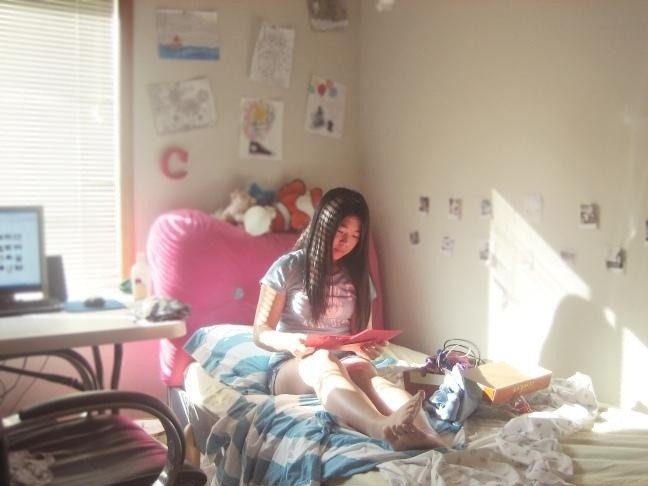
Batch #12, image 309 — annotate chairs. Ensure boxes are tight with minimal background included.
[0,350,207,486]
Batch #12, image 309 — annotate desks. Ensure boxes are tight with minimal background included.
[0,298,187,419]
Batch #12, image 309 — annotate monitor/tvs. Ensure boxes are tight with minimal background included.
[0,206,44,304]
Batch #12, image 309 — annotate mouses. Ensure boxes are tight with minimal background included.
[84,298,105,308]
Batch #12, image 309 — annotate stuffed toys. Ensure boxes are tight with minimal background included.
[216,175,323,235]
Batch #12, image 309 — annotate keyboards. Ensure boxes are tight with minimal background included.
[0,297,64,316]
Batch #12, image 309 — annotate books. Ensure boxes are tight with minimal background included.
[304,325,401,347]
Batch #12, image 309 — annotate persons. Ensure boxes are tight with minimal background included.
[251,187,448,452]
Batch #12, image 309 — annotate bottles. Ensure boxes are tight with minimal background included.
[131,252,150,300]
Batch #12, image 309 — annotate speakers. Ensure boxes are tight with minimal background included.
[45,255,68,304]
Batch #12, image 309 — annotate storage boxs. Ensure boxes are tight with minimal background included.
[403,358,553,405]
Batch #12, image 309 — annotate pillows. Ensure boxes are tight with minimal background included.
[183,322,272,394]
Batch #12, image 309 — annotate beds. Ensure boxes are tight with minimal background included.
[166,341,648,486]
